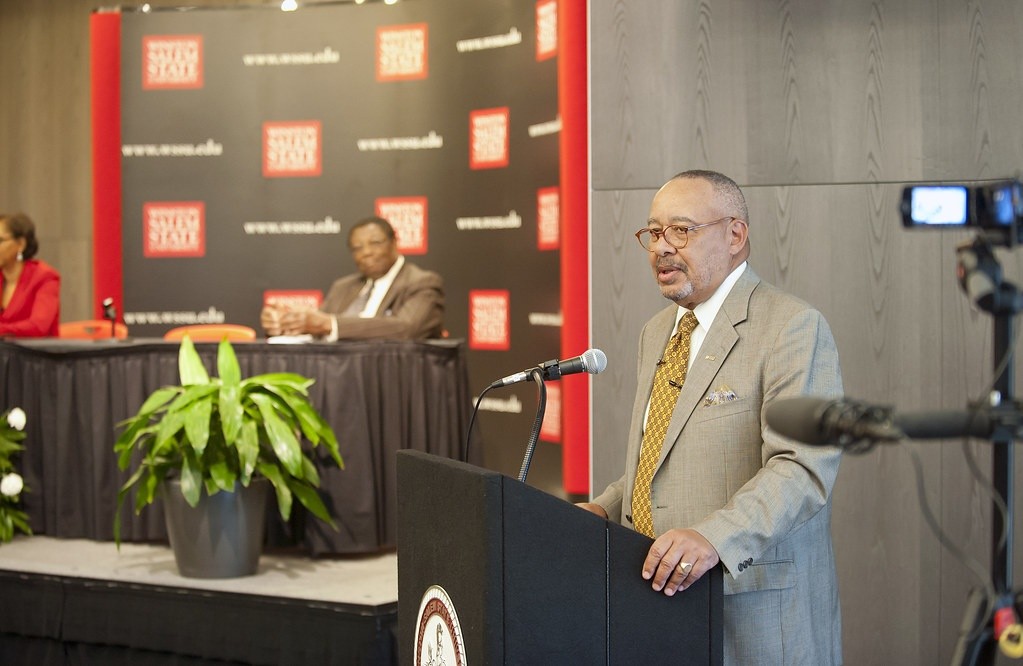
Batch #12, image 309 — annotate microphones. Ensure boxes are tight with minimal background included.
[491,349,608,389]
[766,395,902,447]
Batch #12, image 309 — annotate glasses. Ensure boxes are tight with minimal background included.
[634,216,736,252]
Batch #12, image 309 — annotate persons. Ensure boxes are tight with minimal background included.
[575,170,845,666]
[0,211,61,340]
[260,215,448,344]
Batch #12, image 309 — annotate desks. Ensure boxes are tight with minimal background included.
[1,337,479,563]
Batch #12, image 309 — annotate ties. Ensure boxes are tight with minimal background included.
[631,309,699,540]
[340,277,376,318]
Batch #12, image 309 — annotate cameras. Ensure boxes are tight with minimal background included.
[898,179,1023,230]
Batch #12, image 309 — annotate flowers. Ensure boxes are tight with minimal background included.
[0,407,33,544]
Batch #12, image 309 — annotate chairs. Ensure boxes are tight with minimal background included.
[60,320,128,339]
[166,324,256,341]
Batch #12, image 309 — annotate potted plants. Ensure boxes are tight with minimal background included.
[115,333,344,578]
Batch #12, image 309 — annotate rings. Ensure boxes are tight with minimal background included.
[678,562,692,569]
